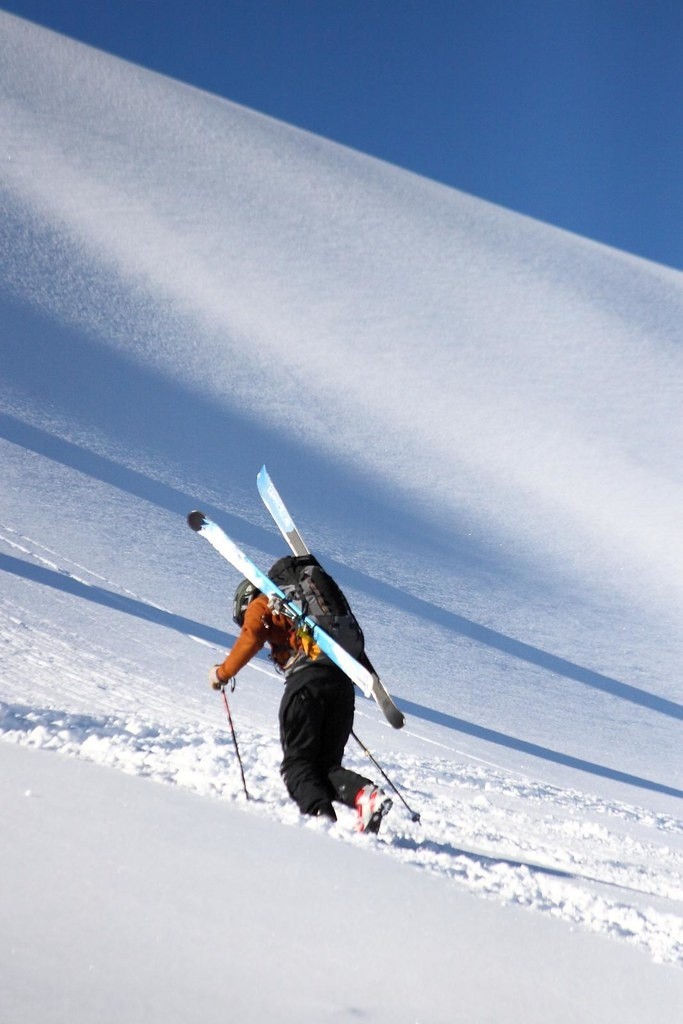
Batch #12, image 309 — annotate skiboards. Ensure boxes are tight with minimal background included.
[186,507,375,701]
[255,463,408,731]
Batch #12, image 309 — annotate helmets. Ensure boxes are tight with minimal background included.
[233,578,262,629]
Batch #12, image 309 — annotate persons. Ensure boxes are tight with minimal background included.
[209,579,393,836]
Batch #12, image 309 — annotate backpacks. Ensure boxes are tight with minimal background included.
[266,554,364,671]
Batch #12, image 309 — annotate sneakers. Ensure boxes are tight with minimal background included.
[354,785,392,835]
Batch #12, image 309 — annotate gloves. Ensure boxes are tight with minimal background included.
[209,665,228,691]
